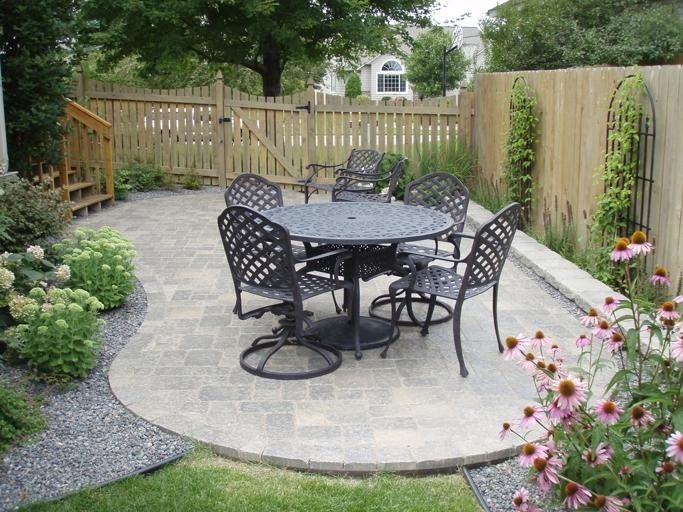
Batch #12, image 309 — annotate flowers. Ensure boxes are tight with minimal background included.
[495,229,683,511]
